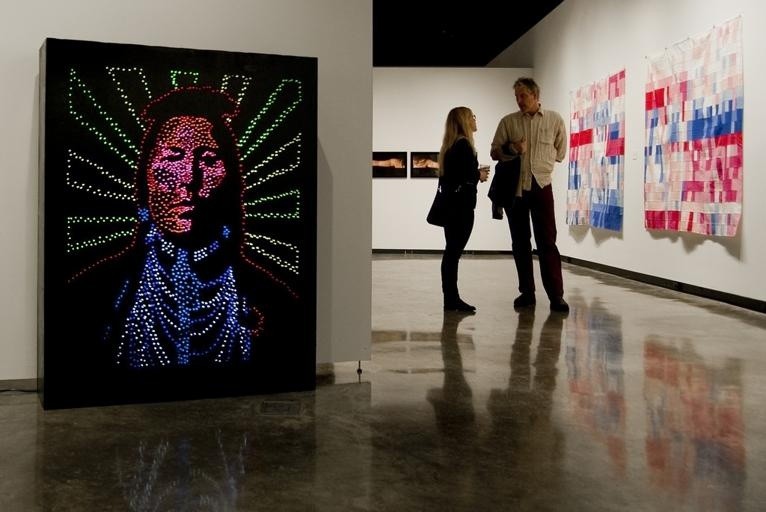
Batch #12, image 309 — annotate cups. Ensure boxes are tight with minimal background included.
[480,164,490,170]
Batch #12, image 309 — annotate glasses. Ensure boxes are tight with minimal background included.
[472,114,476,120]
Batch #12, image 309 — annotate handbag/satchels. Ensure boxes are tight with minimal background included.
[426,188,458,226]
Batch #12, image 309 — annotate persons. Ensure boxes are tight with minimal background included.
[489,78,569,313]
[427,107,490,311]
[94,87,298,403]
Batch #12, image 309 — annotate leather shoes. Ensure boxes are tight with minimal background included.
[550,297,569,313]
[444,293,476,312]
[514,293,536,306]
